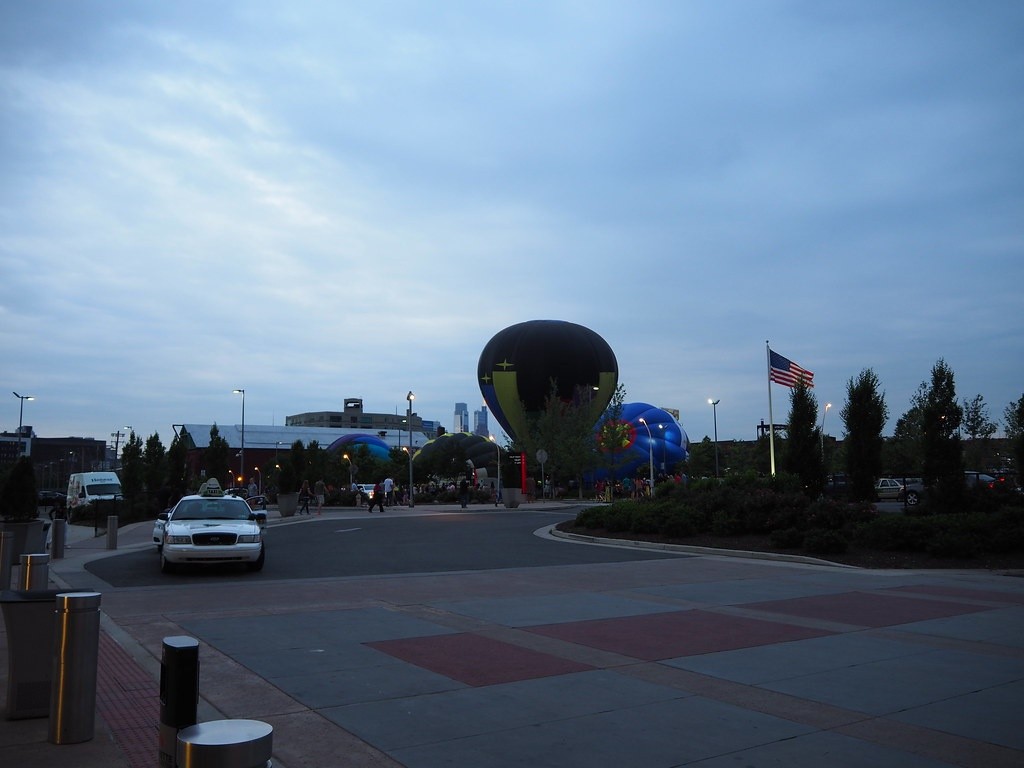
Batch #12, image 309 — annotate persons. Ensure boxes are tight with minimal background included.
[384,475,410,507]
[441,482,456,493]
[49,503,67,522]
[314,475,330,516]
[490,481,496,504]
[595,477,647,501]
[544,475,552,494]
[460,478,470,508]
[352,479,358,491]
[411,481,427,496]
[247,478,258,511]
[298,480,314,515]
[656,473,688,487]
[368,481,385,513]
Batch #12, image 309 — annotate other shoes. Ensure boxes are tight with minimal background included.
[299,512,303,515]
[307,512,311,515]
[380,510,386,512]
[367,509,373,513]
[461,506,468,509]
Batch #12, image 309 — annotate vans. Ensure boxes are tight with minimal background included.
[66,472,127,525]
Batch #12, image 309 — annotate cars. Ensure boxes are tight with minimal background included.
[38,491,68,506]
[152,503,176,550]
[875,479,907,501]
[358,484,388,499]
[965,472,1000,489]
[161,478,269,573]
[223,488,250,498]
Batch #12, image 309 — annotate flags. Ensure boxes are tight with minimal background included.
[770,349,815,390]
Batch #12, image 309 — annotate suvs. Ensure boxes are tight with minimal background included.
[898,479,933,506]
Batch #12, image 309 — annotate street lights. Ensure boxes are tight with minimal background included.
[254,467,261,496]
[228,470,235,494]
[399,419,406,451]
[489,434,501,501]
[708,398,720,478]
[343,454,353,485]
[233,389,246,488]
[658,424,669,477]
[406,391,417,508]
[640,417,654,498]
[12,391,36,462]
[275,441,282,471]
[588,386,600,488]
[821,402,833,468]
